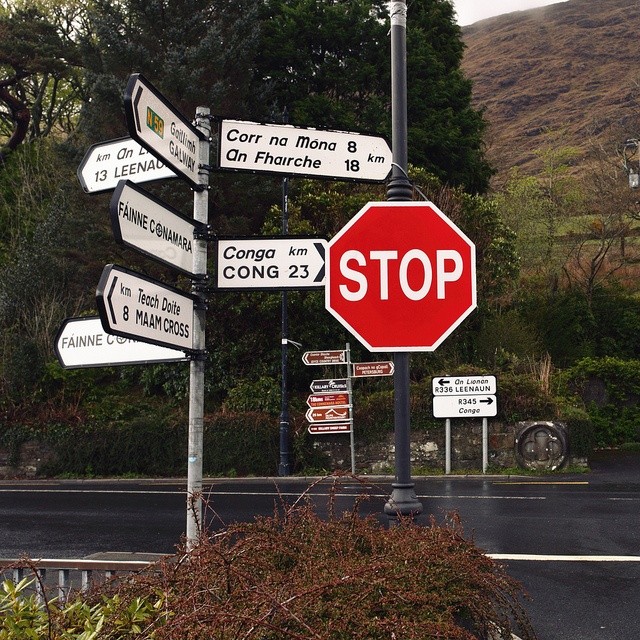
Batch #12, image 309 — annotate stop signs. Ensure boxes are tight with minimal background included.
[325,201,476,353]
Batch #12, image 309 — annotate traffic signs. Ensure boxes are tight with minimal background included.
[96,264,194,352]
[305,409,348,423]
[351,361,395,377]
[77,136,178,194]
[431,375,496,396]
[433,396,497,418]
[306,394,350,409]
[218,119,392,182]
[53,316,189,370]
[216,235,331,291]
[310,379,348,394]
[308,423,350,434]
[123,73,200,191]
[302,350,345,365]
[111,179,193,277]
